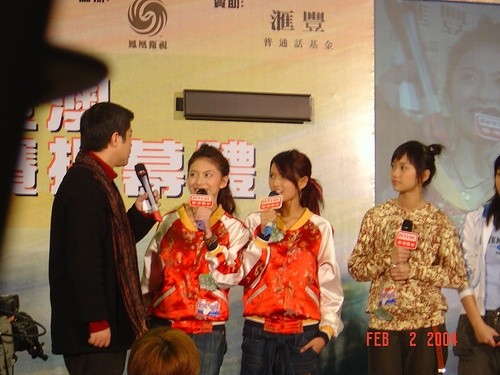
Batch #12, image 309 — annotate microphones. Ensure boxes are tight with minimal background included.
[134,163,162,222]
[189,188,213,231]
[260,191,283,240]
[395,219,418,250]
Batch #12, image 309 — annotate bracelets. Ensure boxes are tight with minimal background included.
[204,234,217,246]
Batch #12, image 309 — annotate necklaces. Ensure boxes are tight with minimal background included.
[450,152,493,201]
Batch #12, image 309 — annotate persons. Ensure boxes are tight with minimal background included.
[421,25,500,226]
[48,101,162,375]
[126,326,200,375]
[237,150,344,375]
[347,140,465,374]
[137,144,253,375]
[453,155,500,375]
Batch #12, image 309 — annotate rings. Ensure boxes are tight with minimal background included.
[394,264,396,269]
